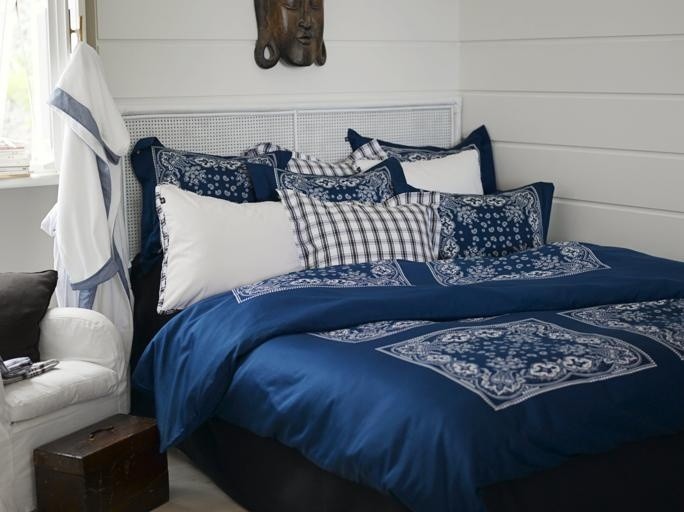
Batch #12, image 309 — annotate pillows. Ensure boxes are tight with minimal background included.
[248,138,389,179]
[0,271,57,363]
[423,181,555,257]
[241,155,410,206]
[367,143,486,196]
[340,122,498,196]
[270,184,445,270]
[131,135,293,269]
[150,181,303,316]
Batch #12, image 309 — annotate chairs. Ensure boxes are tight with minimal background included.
[0,306,125,512]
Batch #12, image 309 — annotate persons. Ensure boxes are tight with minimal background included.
[252,0,328,69]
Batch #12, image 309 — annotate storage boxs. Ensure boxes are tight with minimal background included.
[33,414,169,512]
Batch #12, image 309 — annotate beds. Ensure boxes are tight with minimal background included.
[120,96,684,512]
